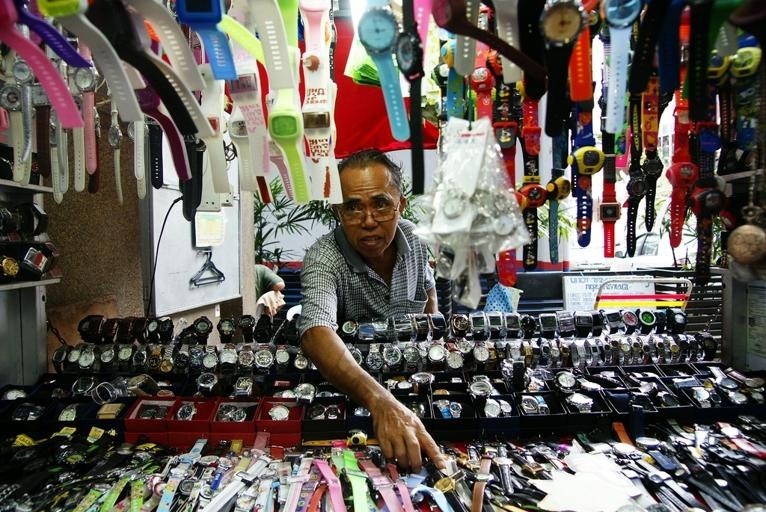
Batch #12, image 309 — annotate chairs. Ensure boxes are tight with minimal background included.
[594,278,693,314]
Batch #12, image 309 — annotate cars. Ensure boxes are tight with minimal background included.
[610,233,658,272]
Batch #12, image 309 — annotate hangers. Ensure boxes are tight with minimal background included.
[191,250,226,289]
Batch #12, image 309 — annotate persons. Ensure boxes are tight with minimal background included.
[298,150,443,474]
[254,263,285,302]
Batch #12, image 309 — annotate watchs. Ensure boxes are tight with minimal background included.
[1,1,766,285]
[1,310,766,512]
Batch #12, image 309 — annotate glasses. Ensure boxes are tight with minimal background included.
[339,201,400,226]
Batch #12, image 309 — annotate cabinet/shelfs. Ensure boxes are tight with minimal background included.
[1,70,62,292]
